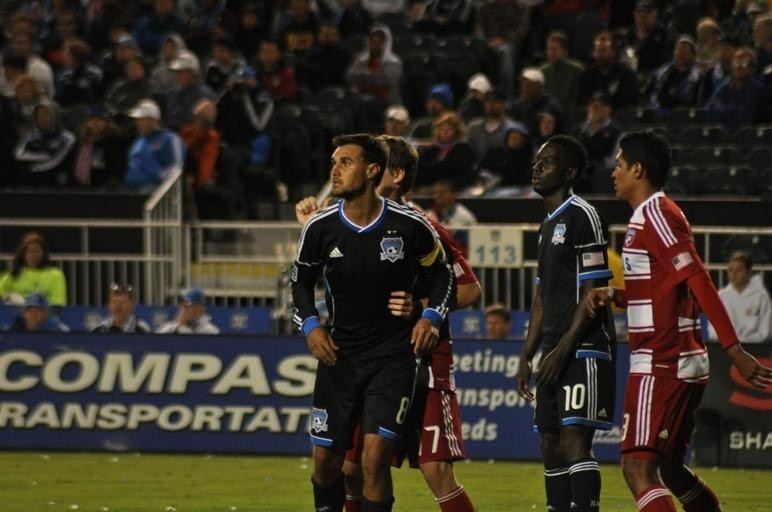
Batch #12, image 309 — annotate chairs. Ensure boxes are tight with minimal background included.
[0,0,770,209]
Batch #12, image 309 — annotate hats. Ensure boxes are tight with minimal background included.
[128,98,160,120]
[169,53,199,72]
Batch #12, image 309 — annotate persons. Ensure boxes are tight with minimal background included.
[2,0,772,228]
[8,293,69,331]
[515,136,616,512]
[486,303,510,338]
[295,135,482,512]
[155,287,220,335]
[88,282,151,334]
[291,133,455,512]
[584,132,771,512]
[2,232,66,305]
[707,251,771,342]
[604,237,625,315]
